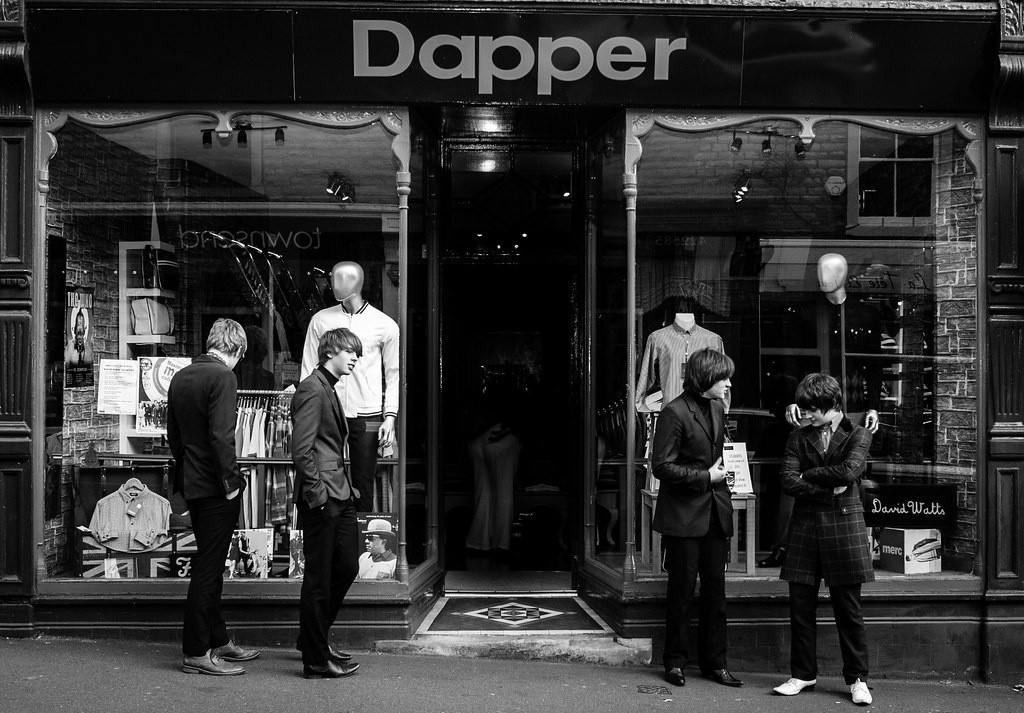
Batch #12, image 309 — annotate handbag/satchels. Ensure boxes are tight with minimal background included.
[142,244,183,291]
[130,297,174,335]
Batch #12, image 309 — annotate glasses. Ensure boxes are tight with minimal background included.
[364,535,378,541]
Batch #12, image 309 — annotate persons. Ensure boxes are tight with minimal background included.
[239,531,255,577]
[636,313,732,415]
[290,533,299,575]
[466,423,523,561]
[772,373,872,704]
[355,519,397,580]
[290,327,359,680]
[299,262,399,511]
[228,531,241,578]
[650,350,742,687]
[167,318,261,674]
[758,332,878,565]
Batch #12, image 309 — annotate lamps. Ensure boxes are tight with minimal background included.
[201,122,288,149]
[730,171,755,203]
[725,126,806,158]
[324,174,356,203]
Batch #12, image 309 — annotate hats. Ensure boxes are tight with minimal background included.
[361,519,395,537]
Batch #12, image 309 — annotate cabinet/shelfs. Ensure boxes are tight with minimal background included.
[117,240,178,453]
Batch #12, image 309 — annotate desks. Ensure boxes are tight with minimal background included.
[640,489,757,576]
[405,486,568,570]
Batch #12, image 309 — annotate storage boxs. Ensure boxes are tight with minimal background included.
[879,527,943,573]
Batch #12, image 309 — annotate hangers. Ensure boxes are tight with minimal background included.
[123,465,144,492]
[237,389,294,411]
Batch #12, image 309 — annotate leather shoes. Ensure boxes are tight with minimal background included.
[774,678,817,696]
[302,656,360,679]
[701,667,744,686]
[849,677,872,705]
[296,641,353,661]
[212,638,260,661]
[182,647,245,676]
[759,549,786,567]
[664,667,686,686]
